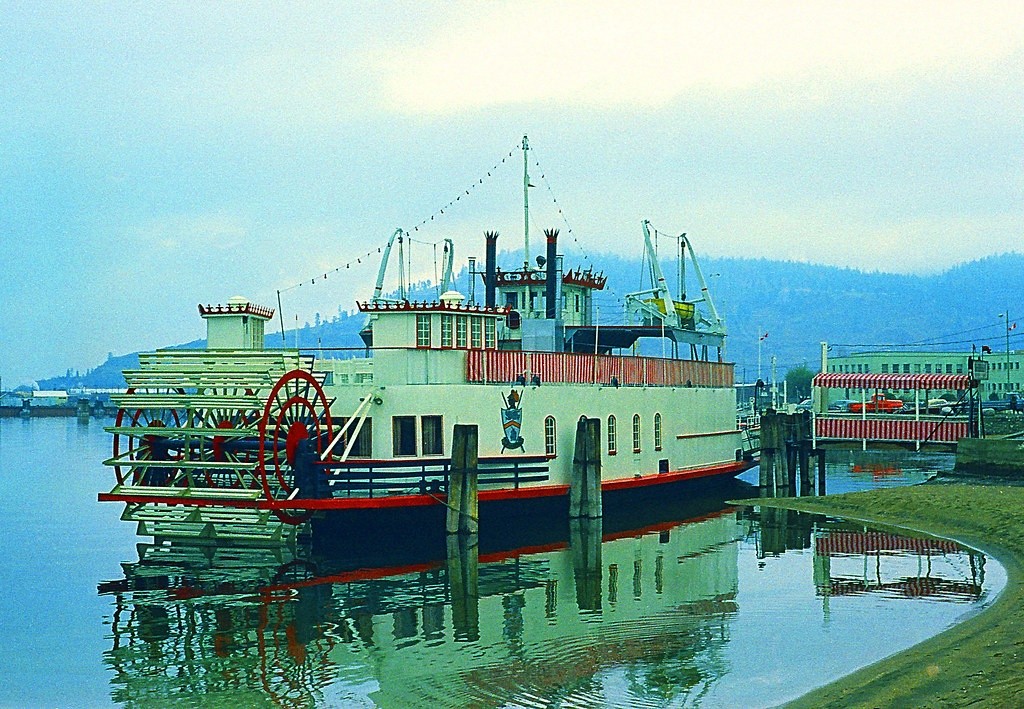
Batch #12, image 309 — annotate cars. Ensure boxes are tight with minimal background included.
[796,399,812,412]
[829,400,856,410]
[847,393,904,413]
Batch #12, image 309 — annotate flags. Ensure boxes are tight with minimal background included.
[982,346,991,354]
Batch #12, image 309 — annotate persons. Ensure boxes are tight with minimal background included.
[1010,396,1019,414]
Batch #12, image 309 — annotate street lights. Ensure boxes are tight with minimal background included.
[998,311,1009,393]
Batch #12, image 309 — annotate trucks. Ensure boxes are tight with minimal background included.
[973,392,1024,414]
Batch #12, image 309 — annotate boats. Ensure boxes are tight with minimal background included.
[96,478,762,709]
[98,136,760,542]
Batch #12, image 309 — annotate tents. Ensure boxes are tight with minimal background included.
[813,522,982,594]
[813,373,970,452]
